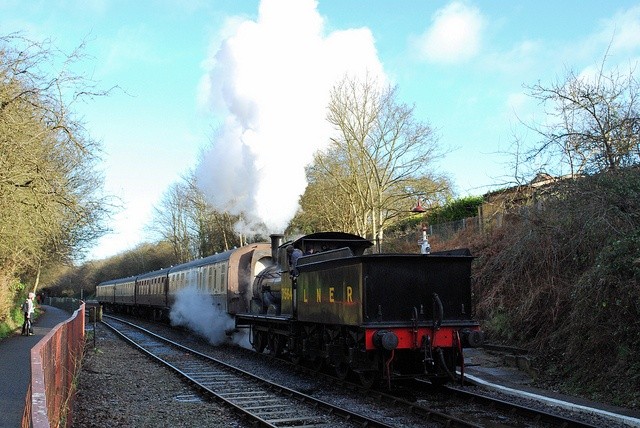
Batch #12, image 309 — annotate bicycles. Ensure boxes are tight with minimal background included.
[20,303,36,336]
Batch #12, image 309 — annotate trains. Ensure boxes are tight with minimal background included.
[95,232,483,394]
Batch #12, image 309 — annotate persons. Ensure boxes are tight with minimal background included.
[41,293,45,305]
[21,293,35,336]
[37,294,40,304]
[286,245,303,280]
[304,245,314,256]
